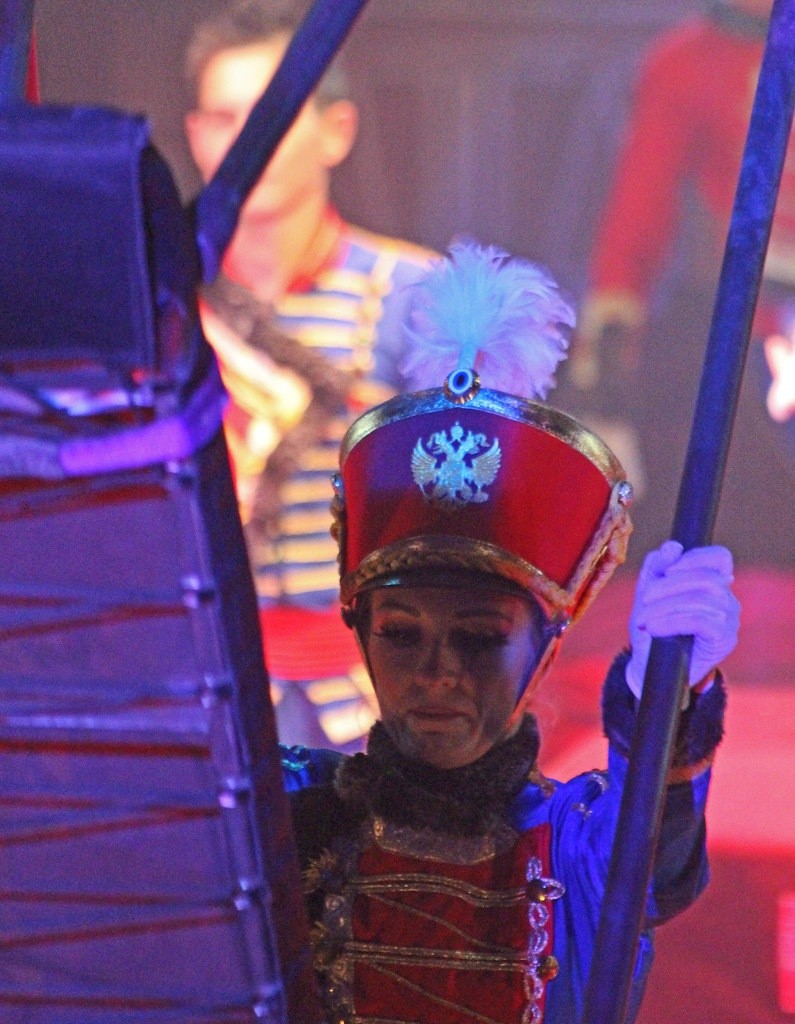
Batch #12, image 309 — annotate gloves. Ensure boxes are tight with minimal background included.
[625,541,742,713]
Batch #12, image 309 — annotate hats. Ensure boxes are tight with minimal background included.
[330,238,634,648]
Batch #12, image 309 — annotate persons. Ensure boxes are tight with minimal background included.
[180,1,477,764]
[280,369,745,1024]
[566,1,794,474]
